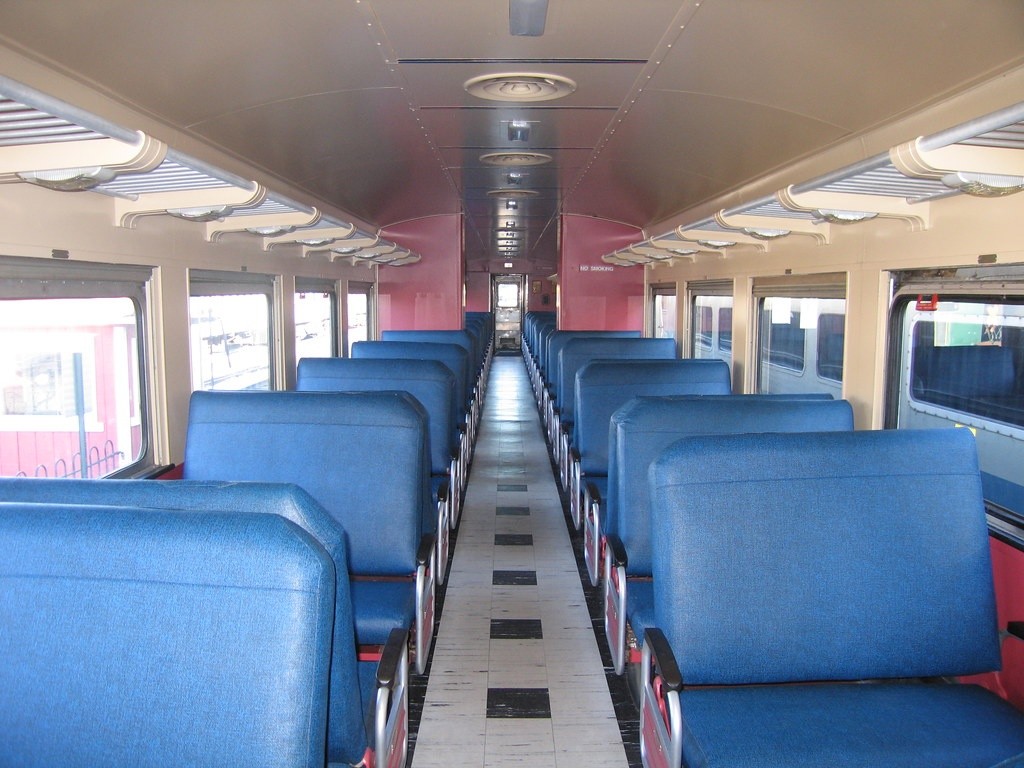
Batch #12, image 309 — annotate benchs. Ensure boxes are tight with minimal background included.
[522,311,1022,766]
[0,312,494,766]
